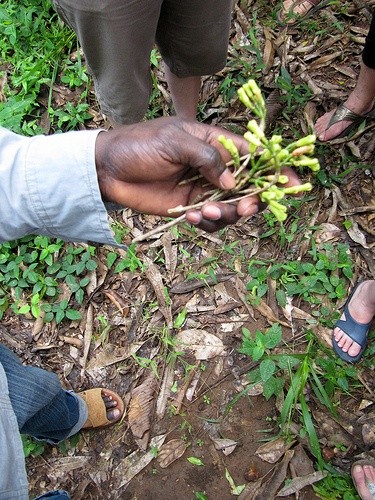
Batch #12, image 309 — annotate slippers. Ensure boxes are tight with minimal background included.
[314,102,375,143]
[332,279,375,365]
[350,459,375,500]
[74,388,125,430]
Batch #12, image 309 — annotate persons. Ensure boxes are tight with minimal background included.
[52,0,235,132]
[331,278,375,364]
[0,115,303,500]
[315,6,375,145]
[351,461,375,500]
[276,0,320,26]
